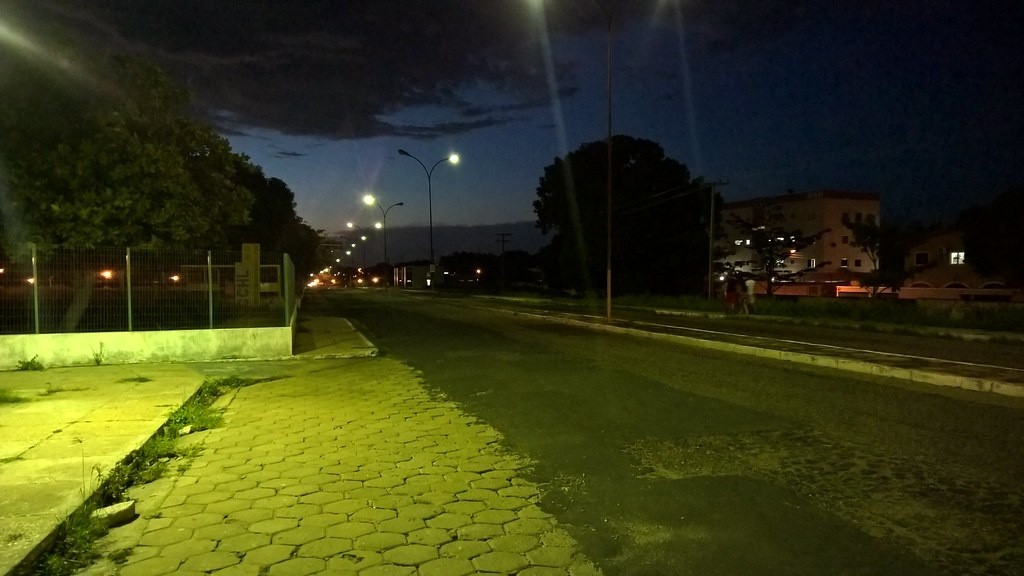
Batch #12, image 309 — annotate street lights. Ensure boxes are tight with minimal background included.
[347,222,382,272]
[364,195,404,272]
[397,149,460,290]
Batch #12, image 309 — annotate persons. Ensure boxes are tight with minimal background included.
[723,272,758,314]
